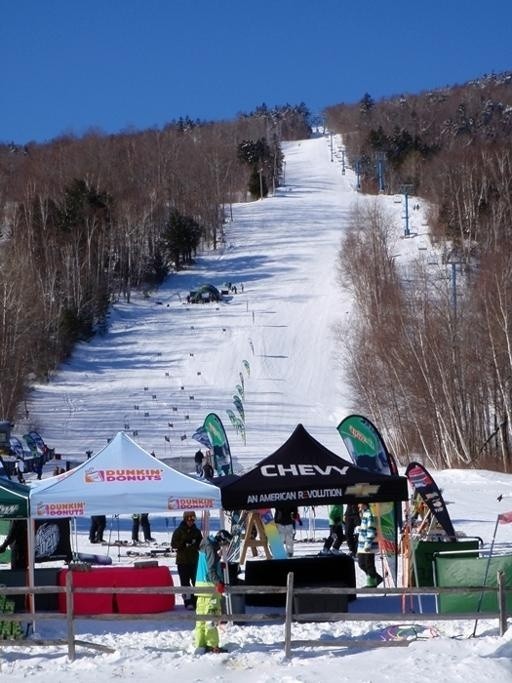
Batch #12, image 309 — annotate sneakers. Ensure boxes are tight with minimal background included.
[132,538,141,543]
[144,537,155,542]
[89,539,106,544]
[196,646,227,653]
[320,548,341,554]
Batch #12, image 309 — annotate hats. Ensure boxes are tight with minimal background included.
[184,511,196,519]
[215,529,233,543]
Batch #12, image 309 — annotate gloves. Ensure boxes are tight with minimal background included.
[215,580,226,594]
[0,544,6,554]
[354,525,361,534]
[291,512,304,525]
[364,541,372,551]
[334,517,342,525]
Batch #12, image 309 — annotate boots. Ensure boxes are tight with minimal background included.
[361,572,384,587]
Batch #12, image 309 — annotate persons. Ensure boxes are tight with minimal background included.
[35,463,43,479]
[195,449,204,473]
[170,510,206,611]
[199,458,214,480]
[1,519,29,572]
[129,512,156,542]
[224,279,245,294]
[344,502,362,557]
[191,528,234,656]
[88,515,108,544]
[292,510,303,541]
[15,456,26,482]
[411,493,430,522]
[319,504,347,554]
[273,501,298,556]
[351,503,384,587]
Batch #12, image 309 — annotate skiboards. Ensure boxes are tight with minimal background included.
[101,540,178,557]
